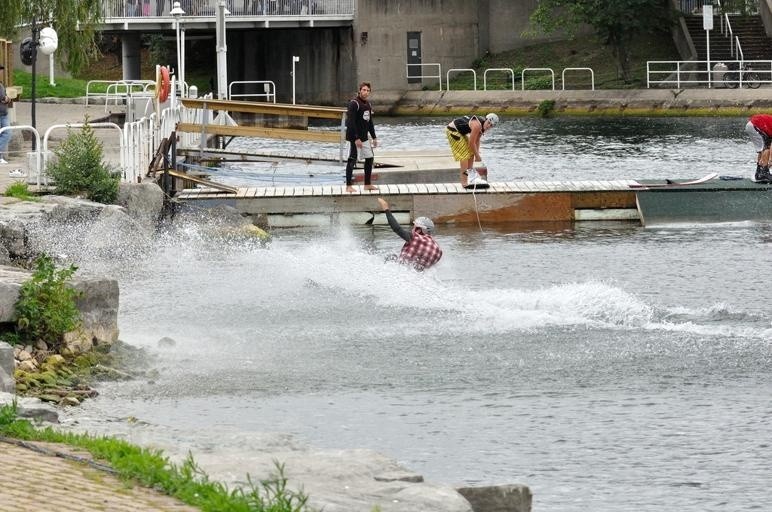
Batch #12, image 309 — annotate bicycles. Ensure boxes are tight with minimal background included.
[723,63,761,89]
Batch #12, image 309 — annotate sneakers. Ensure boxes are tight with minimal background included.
[0,158,9,164]
[7,168,29,180]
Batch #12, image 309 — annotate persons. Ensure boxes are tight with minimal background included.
[375,197,443,273]
[344,81,380,192]
[446,113,499,188]
[0,64,13,162]
[107,1,325,20]
[744,113,771,183]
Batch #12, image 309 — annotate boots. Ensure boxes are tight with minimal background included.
[755,160,771,183]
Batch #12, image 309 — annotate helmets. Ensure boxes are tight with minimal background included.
[412,215,436,237]
[485,112,499,128]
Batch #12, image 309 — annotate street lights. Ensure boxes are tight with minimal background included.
[170,1,186,98]
[20,17,58,150]
